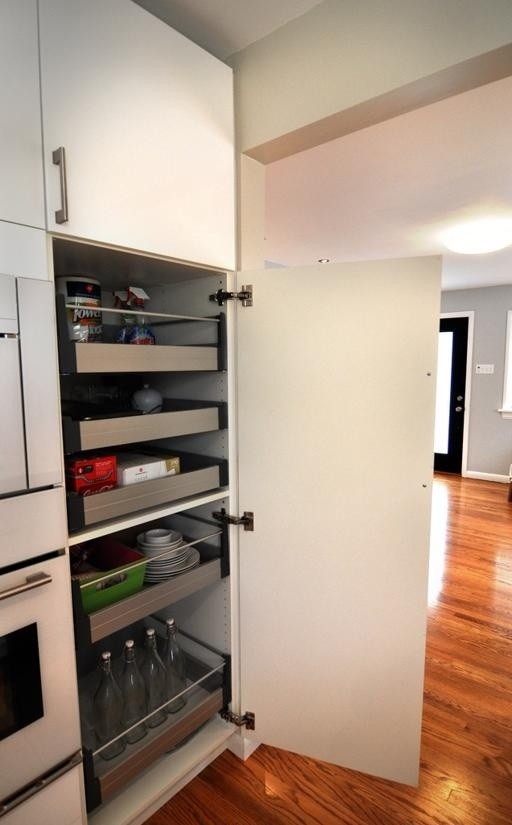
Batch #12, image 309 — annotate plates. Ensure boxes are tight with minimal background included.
[135,528,200,584]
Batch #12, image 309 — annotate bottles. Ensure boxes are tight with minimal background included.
[162,617,187,714]
[92,651,127,760]
[117,639,149,746]
[138,628,170,730]
[133,383,162,415]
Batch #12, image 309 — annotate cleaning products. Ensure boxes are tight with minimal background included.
[112,287,155,347]
